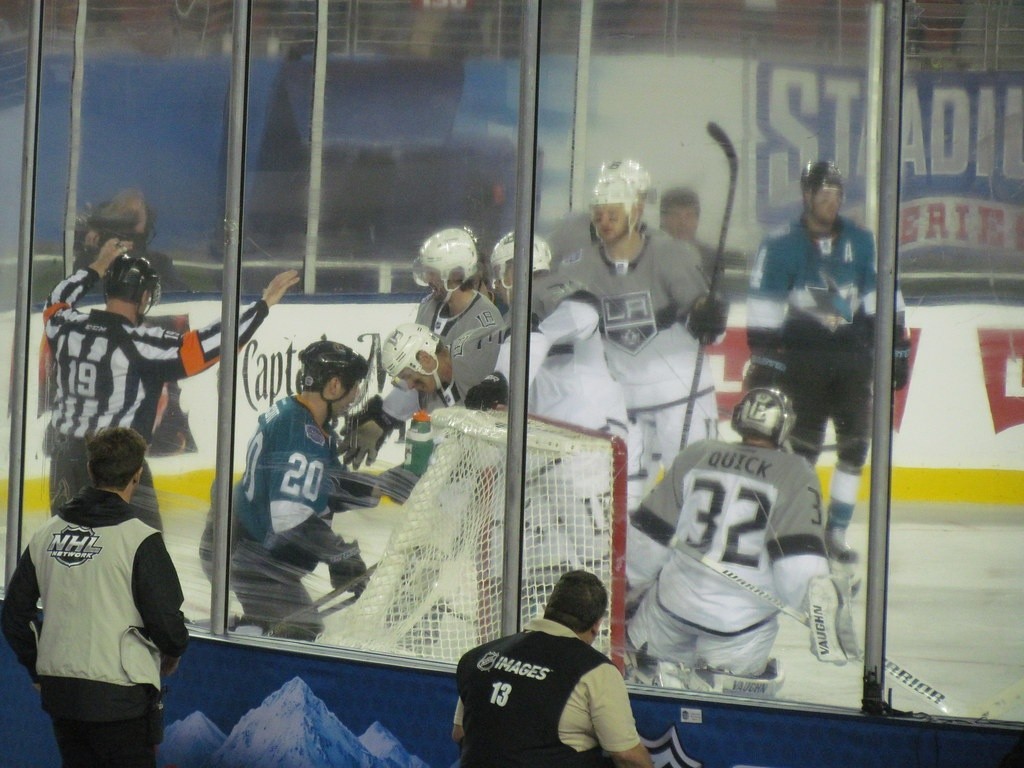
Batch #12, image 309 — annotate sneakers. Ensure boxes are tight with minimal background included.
[825,516,858,562]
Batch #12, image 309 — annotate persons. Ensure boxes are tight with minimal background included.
[555,155,729,504]
[381,228,661,687]
[453,570,653,768]
[744,159,913,576]
[0,427,189,768]
[199,339,434,643]
[42,237,301,543]
[72,187,188,302]
[634,386,860,702]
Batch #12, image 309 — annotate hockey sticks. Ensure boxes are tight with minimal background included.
[371,579,477,624]
[672,539,1023,722]
[678,120,741,450]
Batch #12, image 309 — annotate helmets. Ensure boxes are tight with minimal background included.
[420,229,478,281]
[491,231,551,274]
[802,159,843,190]
[300,341,368,383]
[381,324,441,376]
[590,175,635,213]
[102,253,159,302]
[731,387,795,446]
[601,159,649,190]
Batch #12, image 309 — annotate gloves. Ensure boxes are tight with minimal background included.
[746,326,788,391]
[889,326,912,389]
[327,541,370,598]
[338,395,405,470]
[686,289,729,345]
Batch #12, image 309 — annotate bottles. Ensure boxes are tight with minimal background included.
[403,410,434,476]
